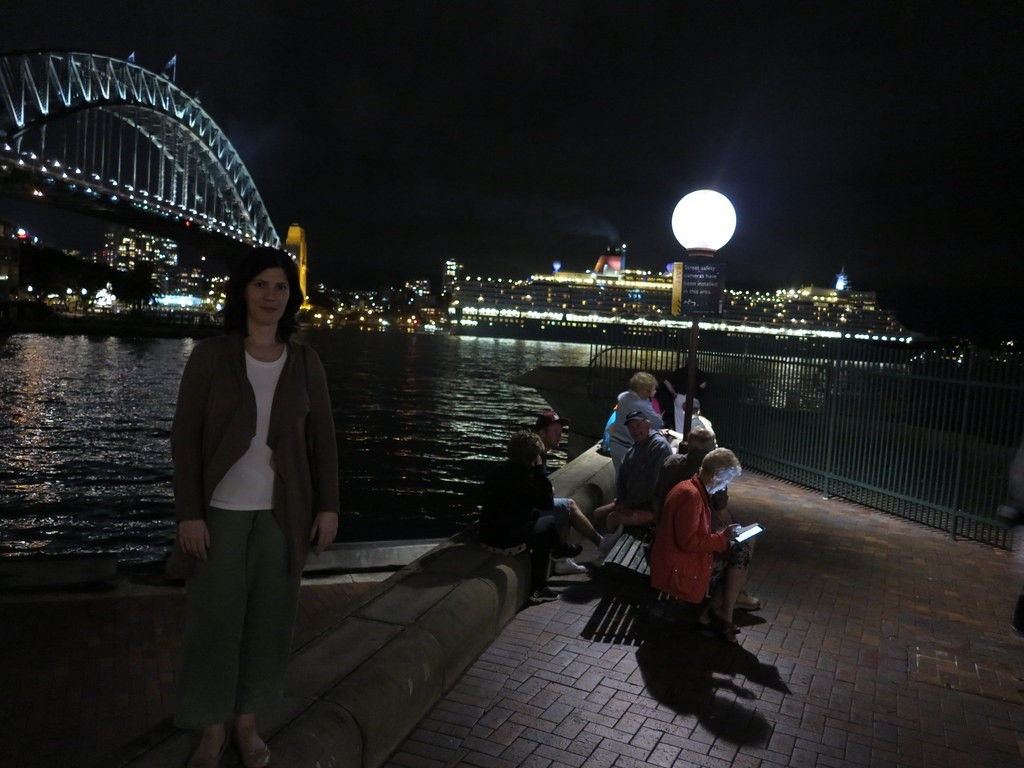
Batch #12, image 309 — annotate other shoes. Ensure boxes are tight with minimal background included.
[549,543,582,561]
[238,742,272,768]
[528,586,558,603]
[553,556,587,574]
[733,592,761,610]
[599,522,624,557]
[187,728,230,768]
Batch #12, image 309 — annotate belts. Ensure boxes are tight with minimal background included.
[676,392,685,395]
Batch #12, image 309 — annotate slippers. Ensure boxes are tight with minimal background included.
[708,605,740,644]
[695,617,717,631]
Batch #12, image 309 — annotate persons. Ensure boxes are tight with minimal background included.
[169,244,342,767]
[478,411,625,604]
[590,355,763,646]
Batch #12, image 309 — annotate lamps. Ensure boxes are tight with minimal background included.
[670,189,738,258]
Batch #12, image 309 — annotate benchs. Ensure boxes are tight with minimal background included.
[601,523,651,599]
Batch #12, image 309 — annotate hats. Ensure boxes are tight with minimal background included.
[683,400,699,410]
[623,411,647,423]
[534,412,572,434]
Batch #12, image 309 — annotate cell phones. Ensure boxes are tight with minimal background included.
[733,525,766,544]
